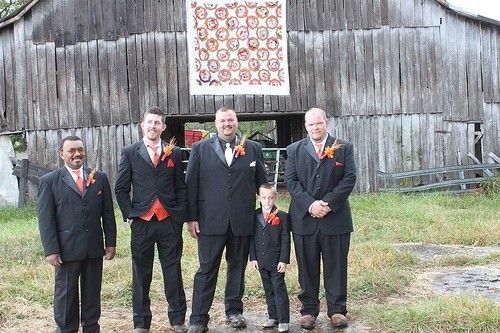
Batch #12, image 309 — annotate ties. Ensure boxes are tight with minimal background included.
[73,170,84,194]
[263,211,269,223]
[224,142,233,166]
[148,145,160,165]
[316,143,323,160]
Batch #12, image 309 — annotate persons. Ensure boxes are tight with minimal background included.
[116,107,188,333]
[248,184,291,333]
[184,107,269,333]
[37,136,117,333]
[283,108,357,330]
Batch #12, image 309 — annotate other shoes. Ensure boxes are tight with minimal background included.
[262,318,278,329]
[330,313,348,328]
[169,323,188,333]
[301,314,316,329]
[187,324,209,333]
[224,313,246,328]
[278,322,289,333]
[133,327,150,333]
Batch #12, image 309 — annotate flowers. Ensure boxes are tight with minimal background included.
[319,138,345,160]
[267,208,280,226]
[234,135,246,158]
[86,168,97,188]
[161,136,177,161]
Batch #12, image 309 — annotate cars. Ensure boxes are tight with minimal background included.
[247,130,286,183]
